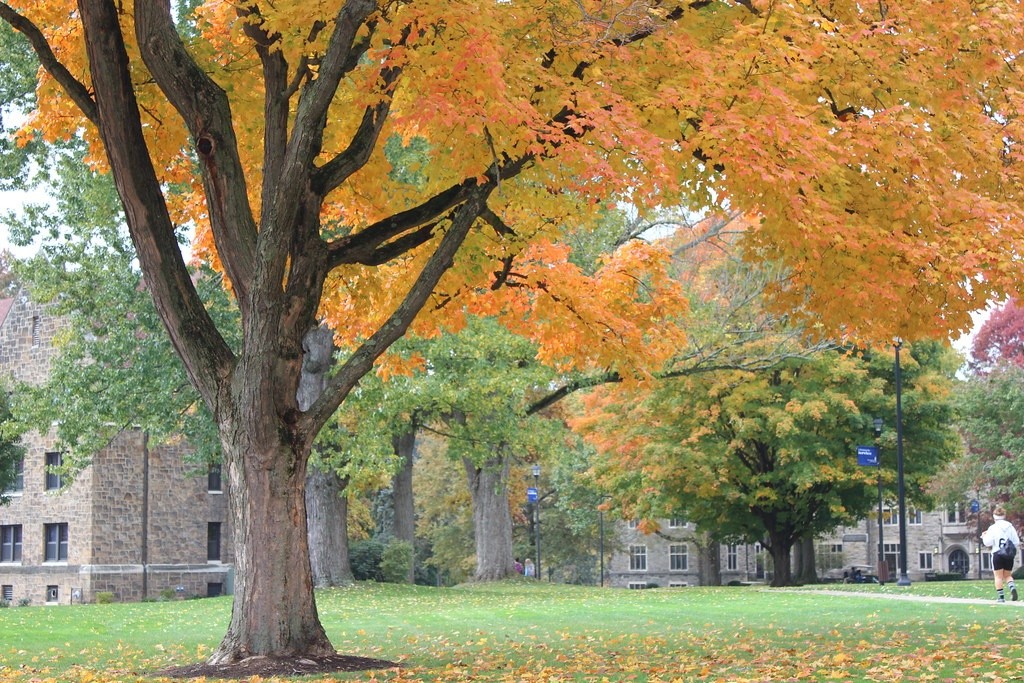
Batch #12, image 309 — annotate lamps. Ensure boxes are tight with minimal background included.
[933,547,938,556]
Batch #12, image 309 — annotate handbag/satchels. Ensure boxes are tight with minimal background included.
[997,538,1017,560]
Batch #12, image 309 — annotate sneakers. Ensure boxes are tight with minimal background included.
[1010,586,1018,601]
[997,593,1006,603]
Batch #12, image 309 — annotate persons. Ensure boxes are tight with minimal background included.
[980,507,1021,602]
[524,558,535,578]
[513,557,523,575]
[843,567,863,584]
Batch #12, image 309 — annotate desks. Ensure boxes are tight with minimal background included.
[862,574,875,583]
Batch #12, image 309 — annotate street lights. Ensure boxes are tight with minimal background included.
[531,464,542,581]
[975,485,981,580]
[872,419,885,586]
